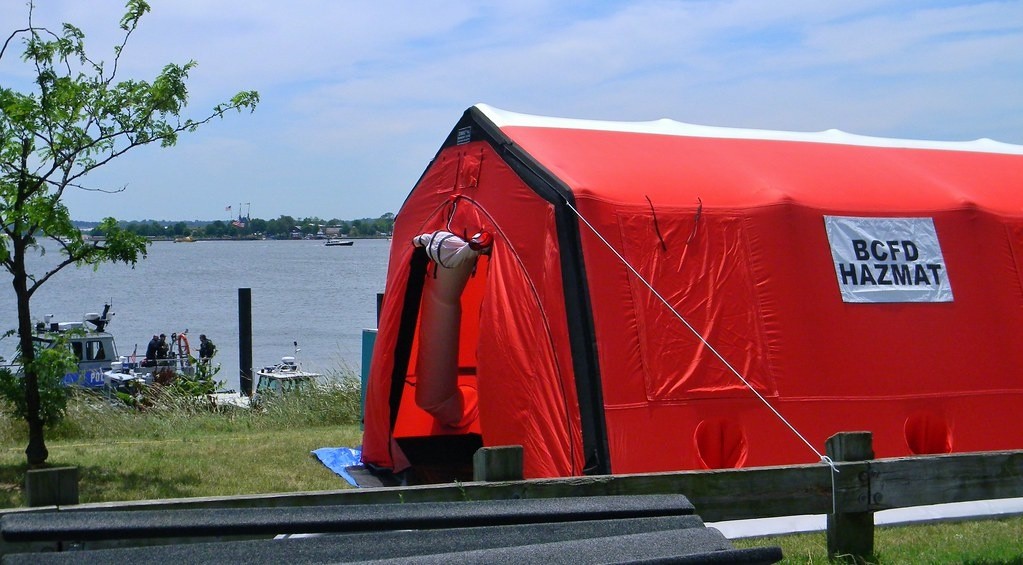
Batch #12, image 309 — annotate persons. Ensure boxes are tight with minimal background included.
[146,333,169,366]
[195,334,213,376]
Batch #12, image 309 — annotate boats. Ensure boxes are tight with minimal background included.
[254,340,362,406]
[0,297,213,394]
[324,238,354,246]
[173,236,196,243]
[62,238,74,247]
[293,233,299,240]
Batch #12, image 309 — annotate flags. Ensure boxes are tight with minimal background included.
[131,346,137,364]
[225,205,232,211]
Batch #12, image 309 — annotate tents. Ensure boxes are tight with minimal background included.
[361,104,1023,480]
